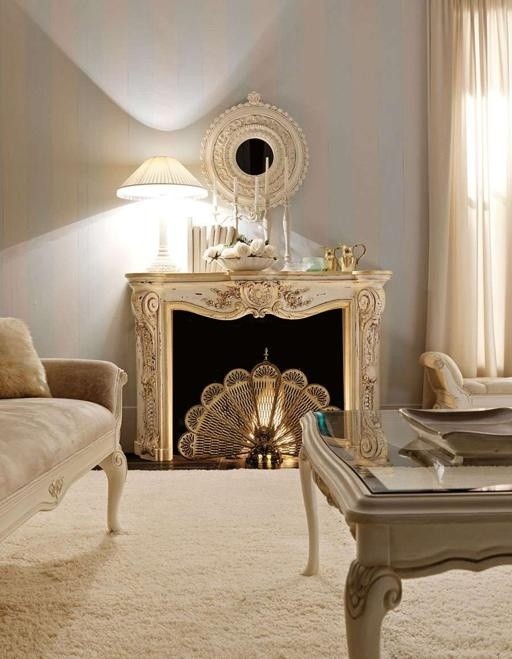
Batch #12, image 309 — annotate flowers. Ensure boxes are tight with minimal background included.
[203,235,279,263]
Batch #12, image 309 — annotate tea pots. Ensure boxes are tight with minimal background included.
[318,243,366,272]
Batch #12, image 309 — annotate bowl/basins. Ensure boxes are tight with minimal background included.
[302,256,324,272]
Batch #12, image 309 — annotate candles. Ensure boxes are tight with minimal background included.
[212,156,289,210]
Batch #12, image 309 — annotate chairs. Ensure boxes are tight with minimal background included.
[1,358,129,547]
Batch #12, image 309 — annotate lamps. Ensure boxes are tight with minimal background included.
[118,156,208,272]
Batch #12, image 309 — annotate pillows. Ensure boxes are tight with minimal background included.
[1,317,53,398]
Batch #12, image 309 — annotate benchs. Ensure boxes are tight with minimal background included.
[418,350,512,409]
